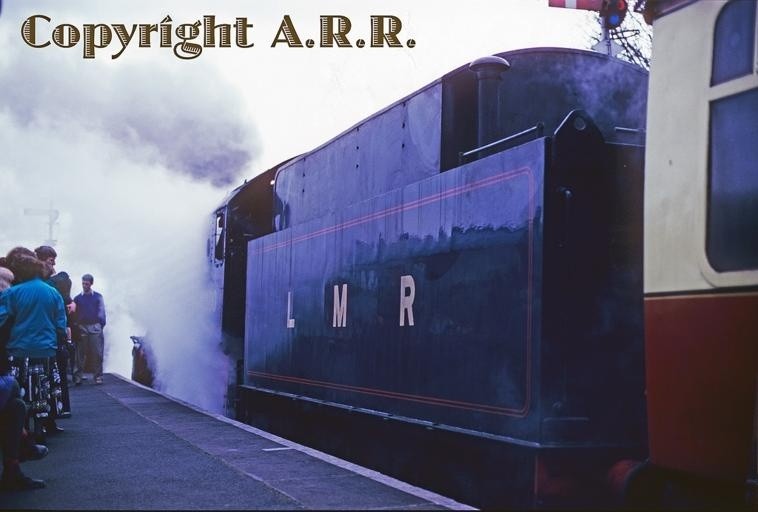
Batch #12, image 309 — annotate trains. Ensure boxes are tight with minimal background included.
[133,1,758,510]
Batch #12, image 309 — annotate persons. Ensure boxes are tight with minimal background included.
[0,244,107,492]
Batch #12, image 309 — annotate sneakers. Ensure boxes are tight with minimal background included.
[21,442,48,462]
[1,469,45,490]
